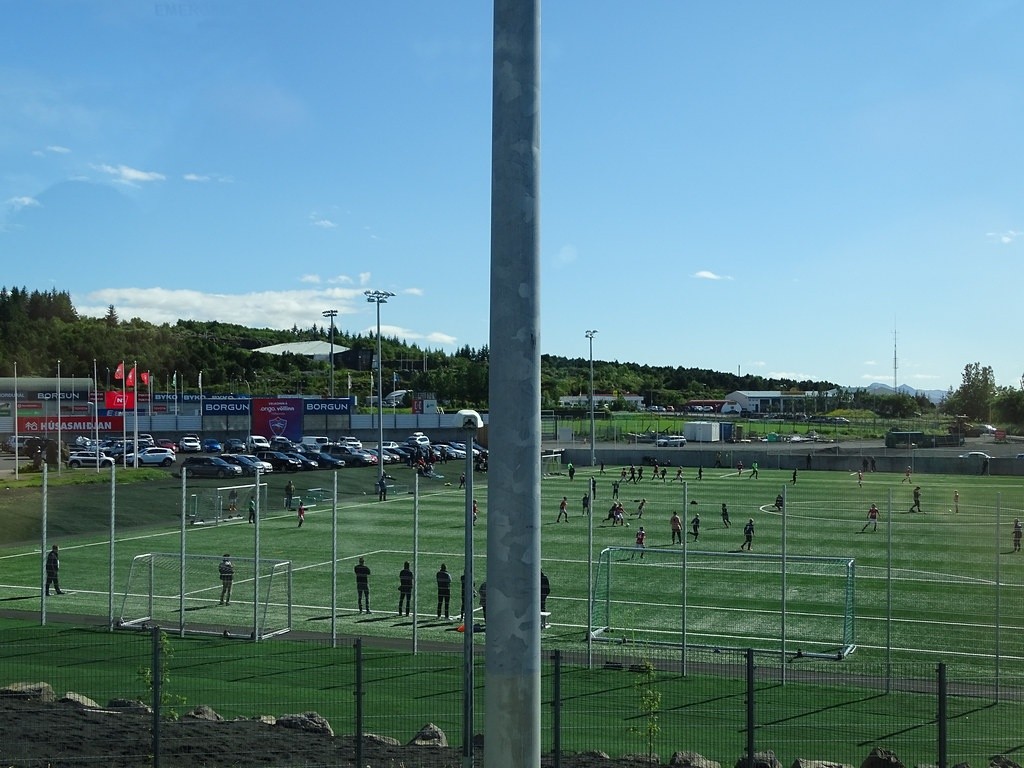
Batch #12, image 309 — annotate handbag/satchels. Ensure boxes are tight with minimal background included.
[220,602,224,604]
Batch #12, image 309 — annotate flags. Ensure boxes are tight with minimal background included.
[126,368,135,387]
[140,373,148,385]
[115,363,123,379]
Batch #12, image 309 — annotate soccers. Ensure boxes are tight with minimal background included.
[625,522,630,526]
[948,510,952,512]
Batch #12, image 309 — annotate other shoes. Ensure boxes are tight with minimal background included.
[366,611,372,614]
[226,602,230,606]
[406,614,409,617]
[399,613,402,616]
[445,617,449,620]
[438,617,441,620]
[360,612,365,615]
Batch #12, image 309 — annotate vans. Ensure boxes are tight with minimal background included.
[5,435,41,455]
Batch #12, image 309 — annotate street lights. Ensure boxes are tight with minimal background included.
[585,329,600,466]
[363,289,396,494]
[322,309,338,398]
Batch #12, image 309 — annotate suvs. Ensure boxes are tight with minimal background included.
[67,451,115,468]
[249,451,303,474]
[179,436,201,454]
[180,456,244,479]
[218,455,264,476]
[655,435,687,447]
[126,447,178,467]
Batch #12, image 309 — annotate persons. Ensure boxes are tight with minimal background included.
[474,452,489,473]
[249,496,256,524]
[379,476,387,501]
[298,502,305,527]
[46,545,66,596]
[473,499,478,526]
[285,481,295,509]
[556,451,1024,560]
[218,554,234,606]
[459,472,466,489]
[229,487,238,510]
[409,445,447,477]
[354,556,550,628]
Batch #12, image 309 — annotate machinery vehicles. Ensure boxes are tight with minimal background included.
[948,416,986,437]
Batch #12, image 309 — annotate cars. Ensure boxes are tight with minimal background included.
[1016,454,1024,458]
[958,451,995,459]
[299,452,346,470]
[975,424,997,434]
[763,412,850,424]
[556,400,609,410]
[246,430,488,470]
[724,432,822,443]
[370,399,401,410]
[637,405,714,413]
[155,438,176,452]
[283,453,319,471]
[67,434,155,462]
[223,439,246,454]
[181,433,200,442]
[238,455,274,474]
[201,439,222,453]
